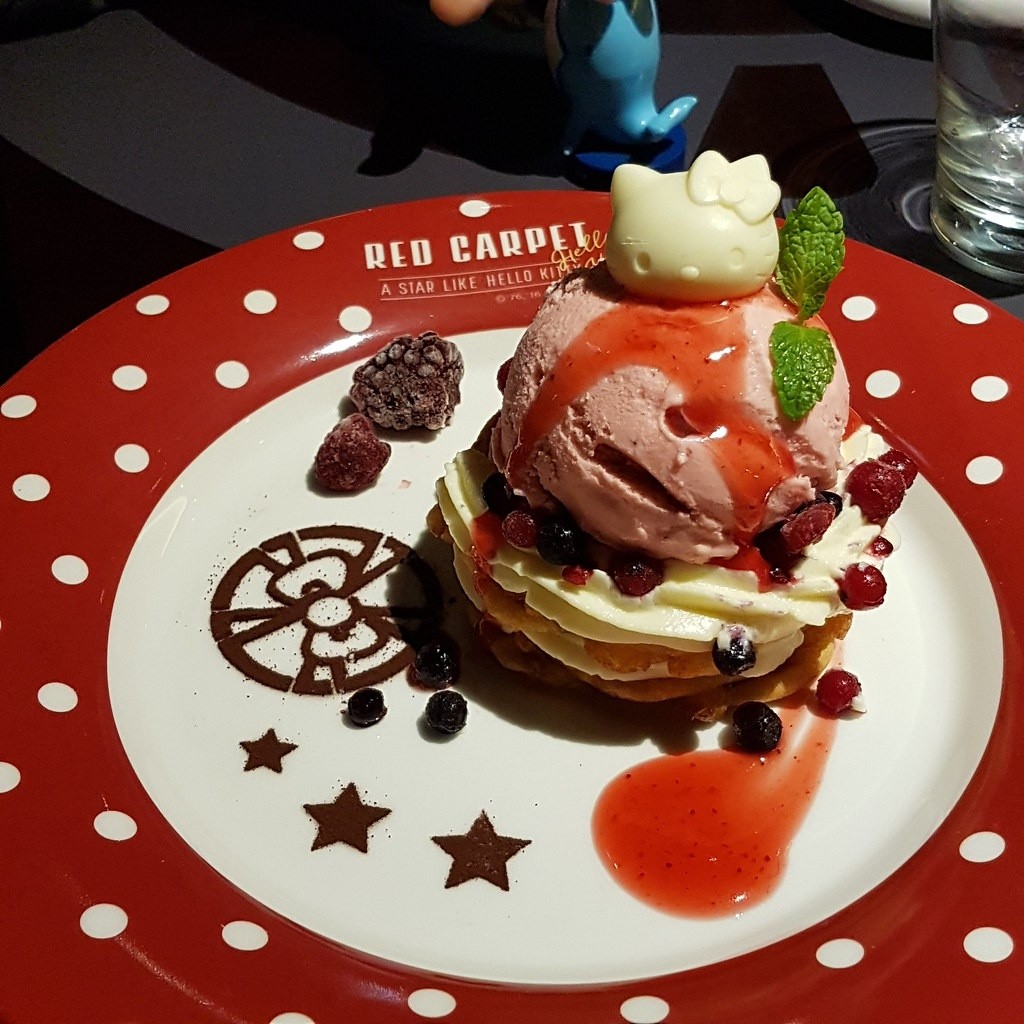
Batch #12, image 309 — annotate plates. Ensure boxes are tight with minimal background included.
[0,189,1024,1024]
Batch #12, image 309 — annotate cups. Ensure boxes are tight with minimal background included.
[930,0,1024,285]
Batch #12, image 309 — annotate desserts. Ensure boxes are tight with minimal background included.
[424,149,919,722]
